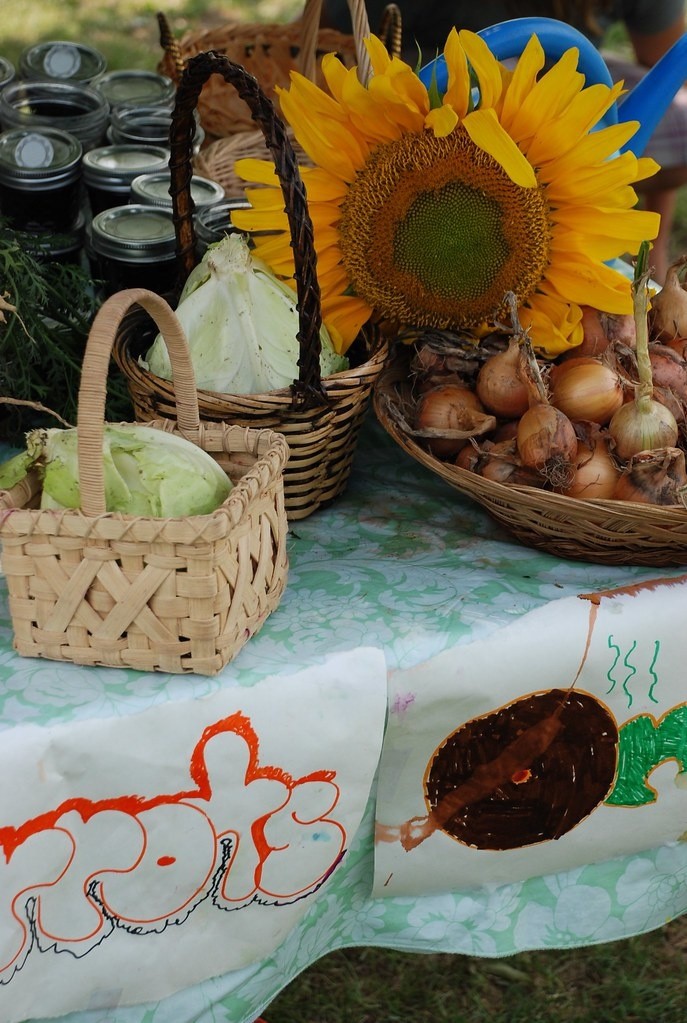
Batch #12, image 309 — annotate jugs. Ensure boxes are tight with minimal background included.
[416,16,687,266]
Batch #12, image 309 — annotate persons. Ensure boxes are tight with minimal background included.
[305,0,687,291]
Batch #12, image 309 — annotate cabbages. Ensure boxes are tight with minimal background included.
[141,231,352,392]
[0,423,234,517]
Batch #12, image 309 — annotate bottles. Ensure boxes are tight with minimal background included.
[0,40,257,296]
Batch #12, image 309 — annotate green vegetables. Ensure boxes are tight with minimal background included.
[0,215,137,448]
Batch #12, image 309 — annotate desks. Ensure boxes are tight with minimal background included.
[0,418,687,1023]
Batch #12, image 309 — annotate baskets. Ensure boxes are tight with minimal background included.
[1,286,287,675]
[371,325,686,571]
[190,0,374,201]
[162,24,370,149]
[111,55,388,523]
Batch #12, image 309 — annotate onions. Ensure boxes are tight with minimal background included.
[407,250,687,506]
